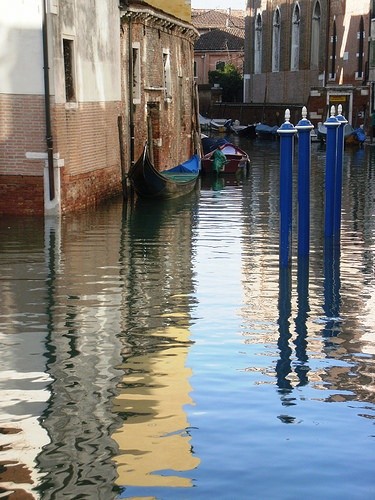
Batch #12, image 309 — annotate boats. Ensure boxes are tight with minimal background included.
[129,148,199,201]
[198,115,320,174]
